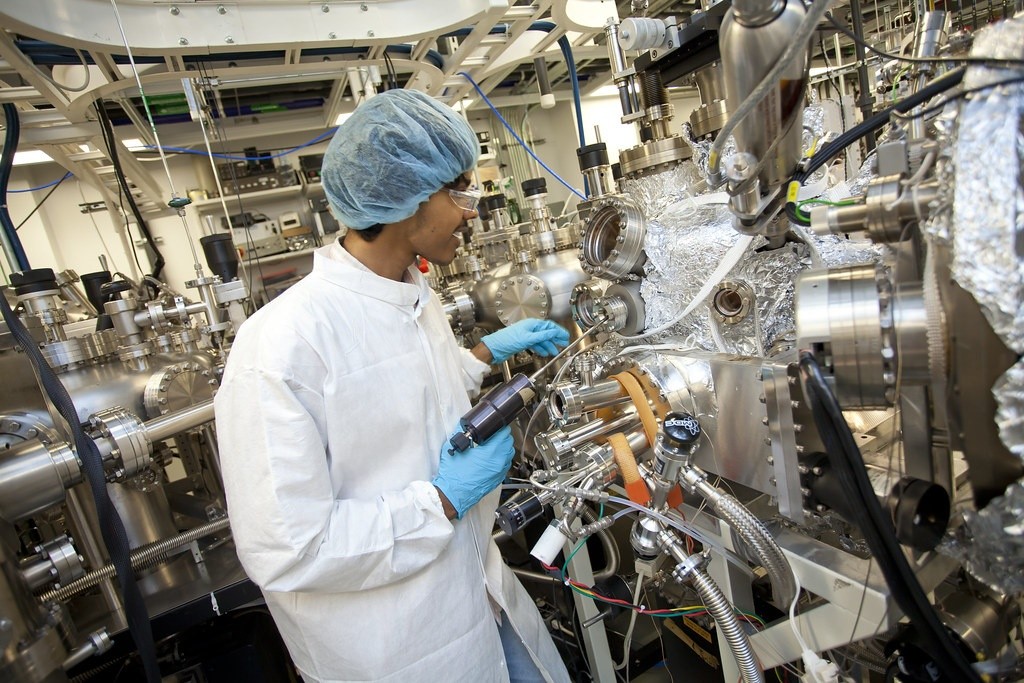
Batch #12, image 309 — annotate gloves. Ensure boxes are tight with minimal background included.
[429,422,516,520]
[481,317,569,365]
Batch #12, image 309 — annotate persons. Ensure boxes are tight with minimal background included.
[214,89,570,683]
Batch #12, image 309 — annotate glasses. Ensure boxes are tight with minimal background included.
[438,167,482,211]
